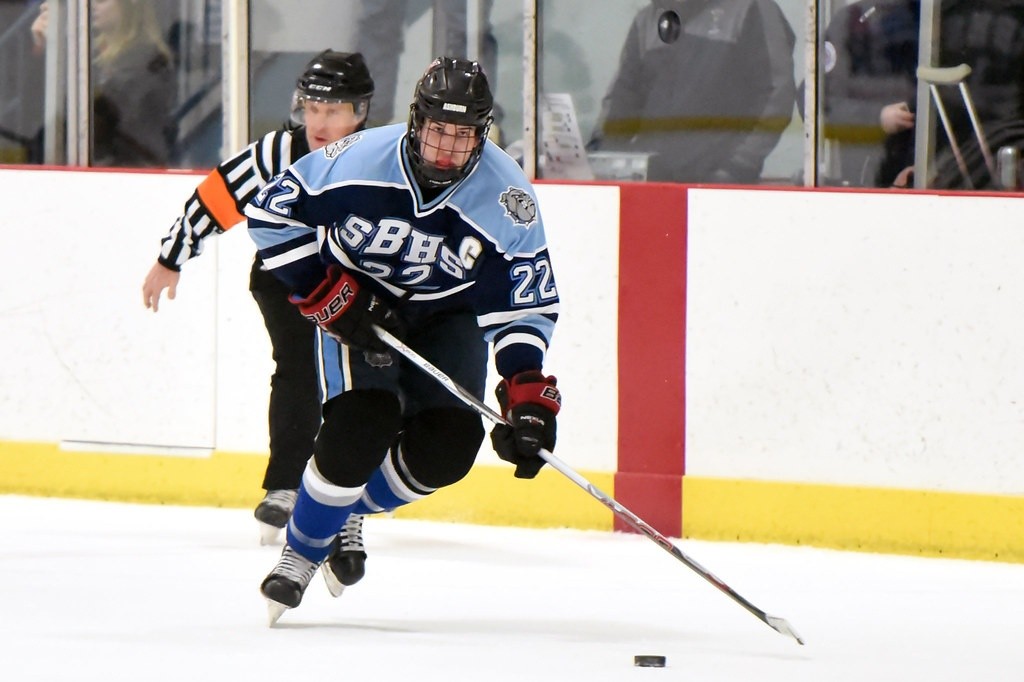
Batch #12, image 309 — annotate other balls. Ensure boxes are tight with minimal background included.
[634,654,666,668]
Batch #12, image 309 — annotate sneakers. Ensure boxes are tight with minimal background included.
[254,489,299,545]
[261,542,322,627]
[321,513,367,598]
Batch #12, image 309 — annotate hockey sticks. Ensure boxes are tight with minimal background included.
[375,328,805,645]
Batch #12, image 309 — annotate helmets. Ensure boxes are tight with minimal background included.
[296,47,374,104]
[406,55,494,188]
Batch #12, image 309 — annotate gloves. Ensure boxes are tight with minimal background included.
[490,369,563,479]
[288,264,403,367]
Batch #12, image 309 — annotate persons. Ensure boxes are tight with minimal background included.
[242,56,563,629]
[31,1,175,166]
[352,0,504,147]
[583,1,797,179]
[794,0,1023,190]
[143,48,376,544]
